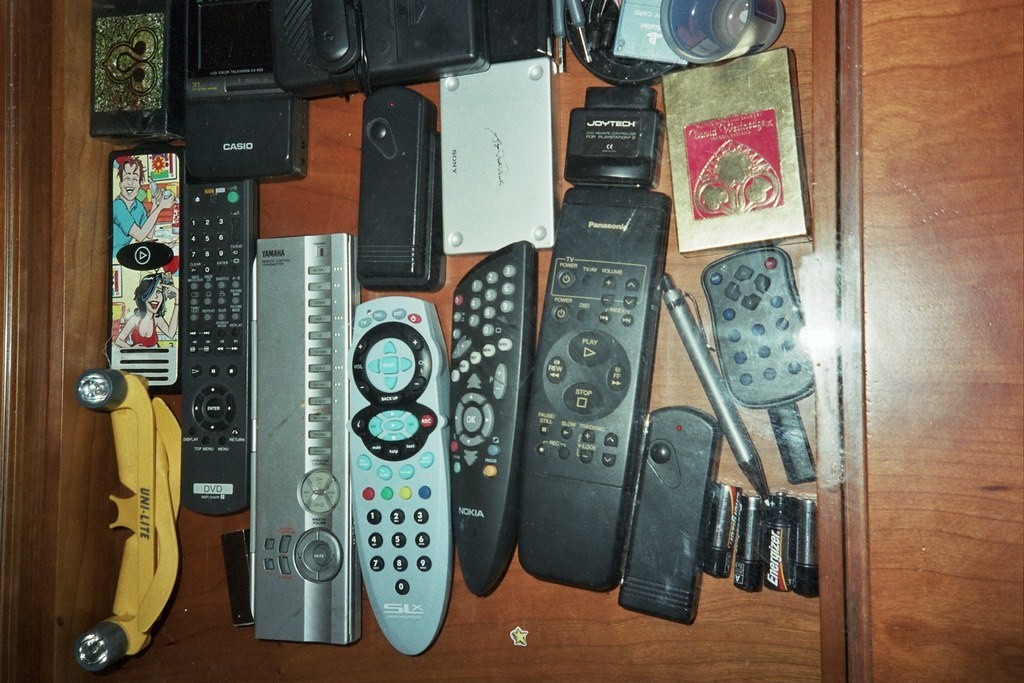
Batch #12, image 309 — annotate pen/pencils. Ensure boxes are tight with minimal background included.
[660,267,775,510]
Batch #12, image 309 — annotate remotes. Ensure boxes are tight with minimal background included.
[701,247,816,409]
[182,178,673,655]
